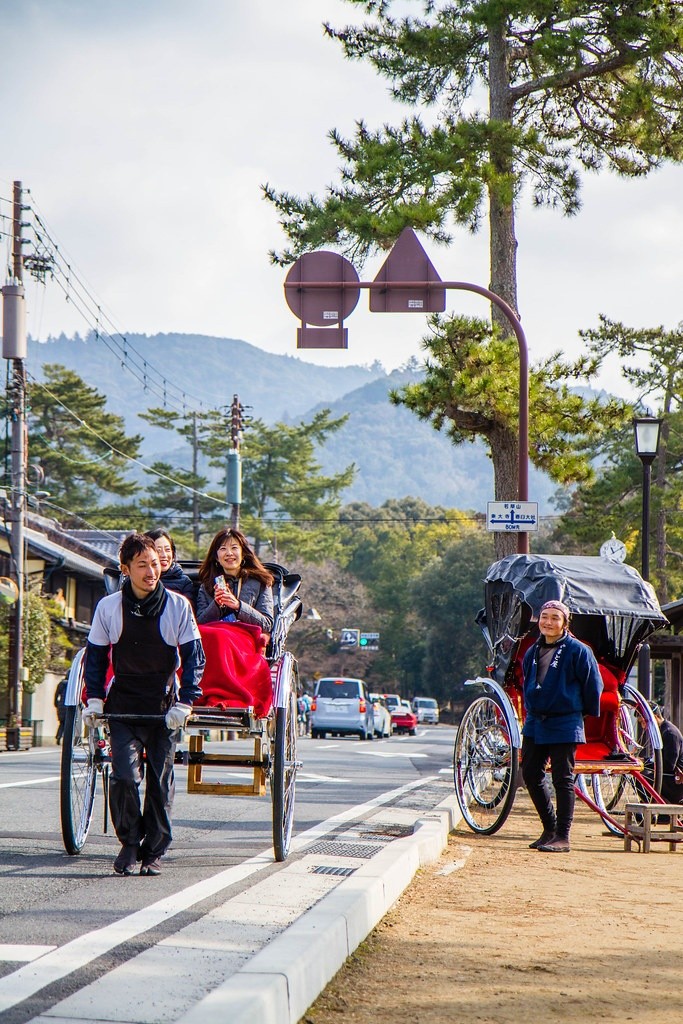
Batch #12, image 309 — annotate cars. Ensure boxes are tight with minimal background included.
[388,706,418,736]
[372,702,392,738]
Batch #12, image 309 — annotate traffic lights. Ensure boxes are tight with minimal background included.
[361,637,379,647]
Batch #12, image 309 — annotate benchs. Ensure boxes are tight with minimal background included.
[513,637,619,713]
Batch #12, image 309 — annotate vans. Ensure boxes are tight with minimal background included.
[412,697,439,725]
[369,693,400,713]
[311,677,375,740]
[401,700,411,708]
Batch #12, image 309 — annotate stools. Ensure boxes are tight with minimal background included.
[188,735,266,797]
[624,803,683,853]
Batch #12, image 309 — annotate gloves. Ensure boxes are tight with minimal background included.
[82,698,105,728]
[164,701,193,730]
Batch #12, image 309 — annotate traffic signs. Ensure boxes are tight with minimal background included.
[487,502,539,532]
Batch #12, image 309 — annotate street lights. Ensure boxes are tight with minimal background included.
[632,406,664,769]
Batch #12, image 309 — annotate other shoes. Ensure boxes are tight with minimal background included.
[537,835,570,852]
[140,857,162,876]
[57,739,60,745]
[113,841,136,875]
[529,831,556,849]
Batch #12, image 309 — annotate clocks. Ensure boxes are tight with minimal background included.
[600,540,627,563]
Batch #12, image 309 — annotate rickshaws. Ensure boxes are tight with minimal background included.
[55,560,304,861]
[453,552,683,852]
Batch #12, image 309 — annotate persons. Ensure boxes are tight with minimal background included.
[82,534,207,876]
[520,600,604,853]
[297,689,313,738]
[196,528,275,633]
[144,528,195,606]
[630,699,683,825]
[54,669,71,745]
